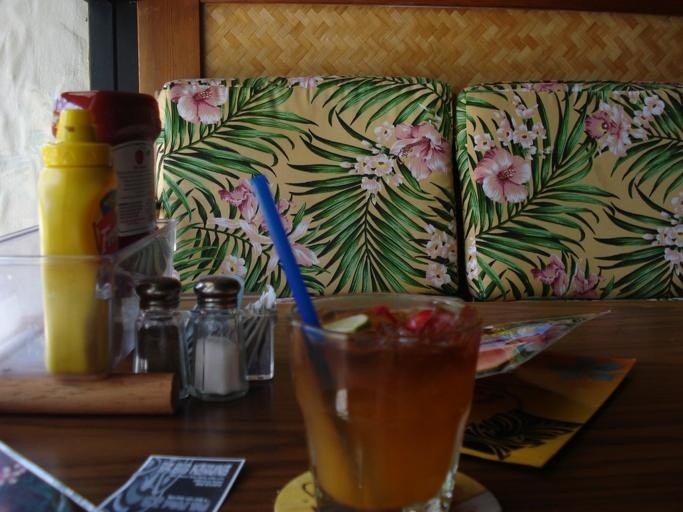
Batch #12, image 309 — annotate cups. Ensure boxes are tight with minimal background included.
[285,291,482,512]
[0,218,179,382]
[136,305,276,384]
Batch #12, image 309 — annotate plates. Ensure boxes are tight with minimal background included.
[273,469,502,512]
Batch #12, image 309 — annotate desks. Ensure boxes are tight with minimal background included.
[0,293,678,509]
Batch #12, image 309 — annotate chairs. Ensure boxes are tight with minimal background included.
[156,68,461,303]
[455,80,682,300]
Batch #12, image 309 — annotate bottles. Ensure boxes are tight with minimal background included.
[37,109,120,374]
[185,277,249,399]
[134,277,185,375]
[51,88,164,357]
